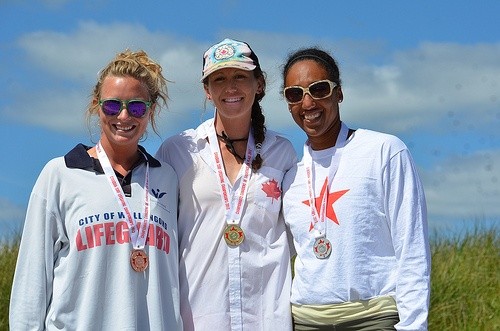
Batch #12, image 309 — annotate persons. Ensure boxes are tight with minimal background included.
[9,50,191,331]
[156,38,302,331]
[275,46,433,331]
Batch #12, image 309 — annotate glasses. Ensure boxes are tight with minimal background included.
[94,98,153,120]
[282,80,337,105]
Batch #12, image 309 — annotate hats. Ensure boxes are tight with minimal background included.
[197,37,263,82]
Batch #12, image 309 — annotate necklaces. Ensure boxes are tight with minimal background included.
[216,130,249,160]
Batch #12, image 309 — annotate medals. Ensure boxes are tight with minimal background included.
[129,248,150,271]
[224,222,245,248]
[313,238,332,260]
